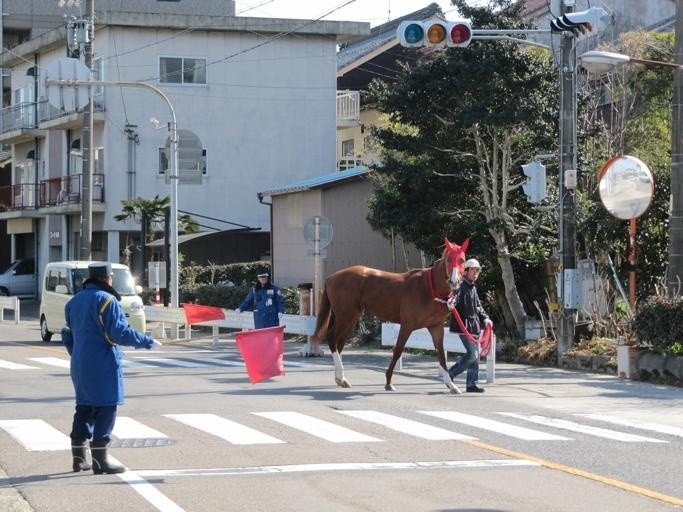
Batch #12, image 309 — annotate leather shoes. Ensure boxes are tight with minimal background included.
[467,386,482,392]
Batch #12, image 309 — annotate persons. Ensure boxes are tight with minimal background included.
[61,263,162,474]
[234,269,284,330]
[447,258,493,392]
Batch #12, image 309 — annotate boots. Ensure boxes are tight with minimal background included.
[71,440,92,472]
[88,442,125,474]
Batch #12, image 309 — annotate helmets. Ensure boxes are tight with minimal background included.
[466,259,481,270]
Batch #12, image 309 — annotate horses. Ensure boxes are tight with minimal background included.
[308,236,471,395]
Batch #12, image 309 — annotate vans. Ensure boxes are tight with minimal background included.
[0,258,36,300]
[39,255,148,350]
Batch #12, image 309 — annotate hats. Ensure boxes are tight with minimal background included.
[89,263,113,279]
[256,269,269,277]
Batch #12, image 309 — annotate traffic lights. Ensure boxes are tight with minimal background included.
[518,158,546,205]
[395,19,473,48]
[548,5,607,45]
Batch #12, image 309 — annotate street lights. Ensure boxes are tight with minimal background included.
[577,51,682,75]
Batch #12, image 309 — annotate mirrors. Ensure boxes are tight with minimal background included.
[596,155,654,220]
